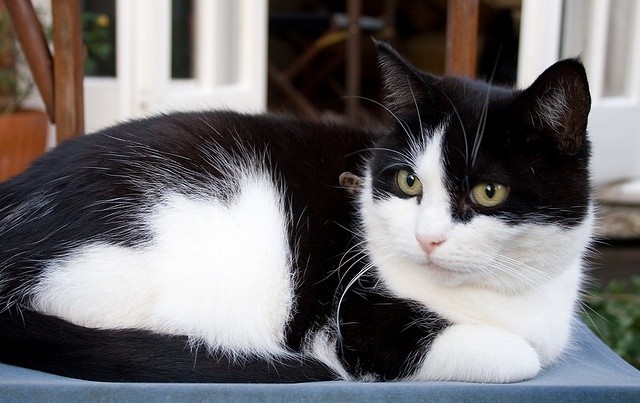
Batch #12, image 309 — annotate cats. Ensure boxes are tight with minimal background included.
[0,34,616,386]
